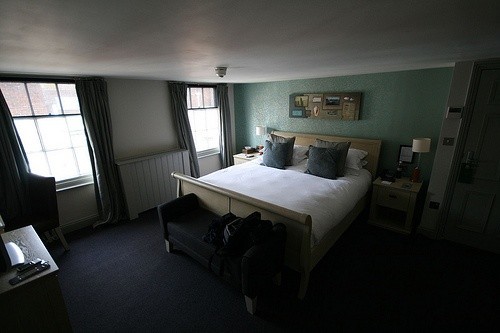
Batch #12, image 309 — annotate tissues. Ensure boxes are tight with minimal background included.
[242,147,253,154]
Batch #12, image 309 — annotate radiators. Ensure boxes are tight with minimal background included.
[117,149,192,220]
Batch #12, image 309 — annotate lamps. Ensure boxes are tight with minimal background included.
[410,138,431,183]
[256,126,264,136]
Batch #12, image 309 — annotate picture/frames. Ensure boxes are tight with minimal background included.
[397,145,415,166]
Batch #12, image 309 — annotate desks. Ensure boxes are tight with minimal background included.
[0,225,73,332]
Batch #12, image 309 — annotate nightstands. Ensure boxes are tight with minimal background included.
[372,175,424,234]
[233,152,261,165]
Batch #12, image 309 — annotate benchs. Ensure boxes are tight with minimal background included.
[158,193,297,316]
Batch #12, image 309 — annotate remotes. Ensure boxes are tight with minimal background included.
[9,262,50,286]
[16,258,42,272]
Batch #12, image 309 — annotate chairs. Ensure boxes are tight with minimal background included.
[24,172,71,251]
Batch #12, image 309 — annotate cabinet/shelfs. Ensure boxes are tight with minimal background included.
[289,91,362,120]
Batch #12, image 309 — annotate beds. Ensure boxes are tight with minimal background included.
[172,130,382,284]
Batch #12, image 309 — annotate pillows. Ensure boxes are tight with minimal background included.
[291,145,310,166]
[345,148,368,170]
[271,134,296,166]
[305,145,340,180]
[260,140,289,169]
[314,138,350,178]
[344,160,368,177]
